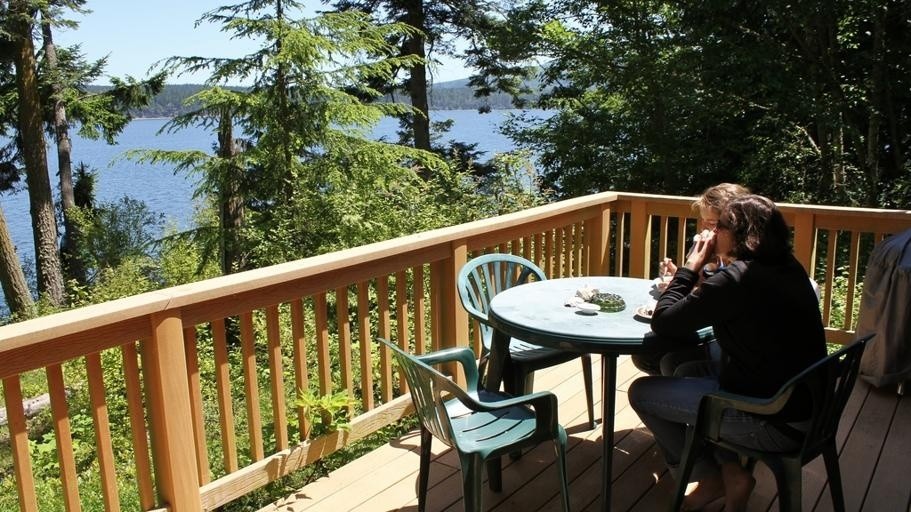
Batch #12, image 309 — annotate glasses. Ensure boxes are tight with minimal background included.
[716,220,729,231]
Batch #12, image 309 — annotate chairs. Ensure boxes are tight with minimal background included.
[373,338,570,512]
[669,331,879,510]
[455,254,596,460]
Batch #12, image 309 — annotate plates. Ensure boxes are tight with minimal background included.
[654,274,674,288]
[636,304,653,321]
[578,303,601,313]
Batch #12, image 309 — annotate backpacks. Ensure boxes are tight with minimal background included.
[856,227,911,393]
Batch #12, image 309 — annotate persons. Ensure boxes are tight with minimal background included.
[627,193,828,511]
[631,182,751,377]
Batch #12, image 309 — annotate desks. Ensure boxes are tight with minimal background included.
[484,274,713,511]
